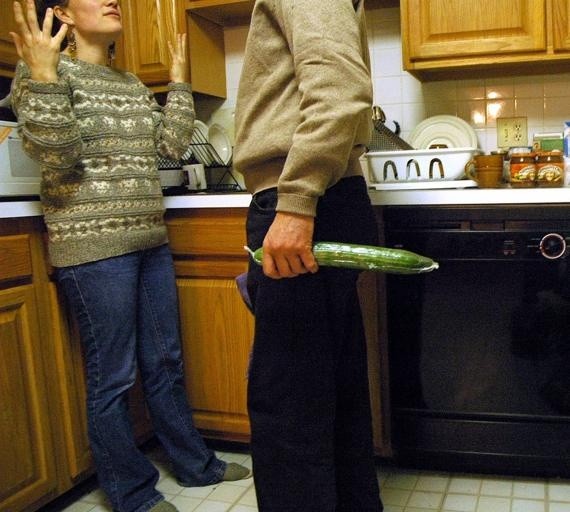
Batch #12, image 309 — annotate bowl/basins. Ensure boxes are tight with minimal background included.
[192,120,231,165]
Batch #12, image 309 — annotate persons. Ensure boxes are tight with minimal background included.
[229,0,381,511]
[8,1,250,512]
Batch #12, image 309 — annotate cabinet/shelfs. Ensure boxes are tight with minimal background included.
[0,207,383,512]
[0,0,570,102]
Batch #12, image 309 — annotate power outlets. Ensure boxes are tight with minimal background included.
[496,116,529,147]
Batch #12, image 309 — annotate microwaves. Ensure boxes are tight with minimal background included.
[0,121,43,202]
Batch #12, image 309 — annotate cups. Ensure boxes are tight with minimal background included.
[181,163,207,191]
[466,153,504,190]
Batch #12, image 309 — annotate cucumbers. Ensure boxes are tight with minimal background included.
[252,241,439,275]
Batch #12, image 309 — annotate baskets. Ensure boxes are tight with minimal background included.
[366,147,485,183]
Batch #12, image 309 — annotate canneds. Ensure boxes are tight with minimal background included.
[510,152,537,188]
[538,151,564,188]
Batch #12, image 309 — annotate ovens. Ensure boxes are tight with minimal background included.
[372,205,570,481]
